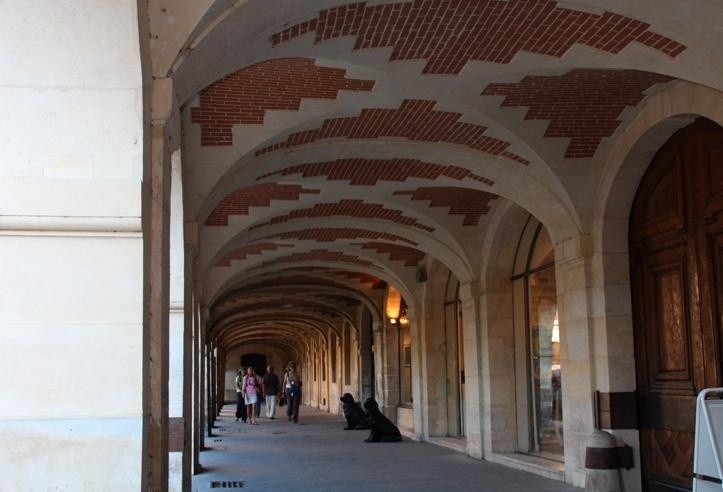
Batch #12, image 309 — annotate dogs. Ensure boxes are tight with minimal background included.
[338,392,371,430]
[362,396,402,442]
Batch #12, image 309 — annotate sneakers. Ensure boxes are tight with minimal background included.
[235,415,298,425]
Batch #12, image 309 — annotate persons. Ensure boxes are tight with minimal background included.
[281,361,302,423]
[234,365,264,426]
[261,365,280,419]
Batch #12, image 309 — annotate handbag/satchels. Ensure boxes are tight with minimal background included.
[292,385,302,396]
[255,383,262,396]
